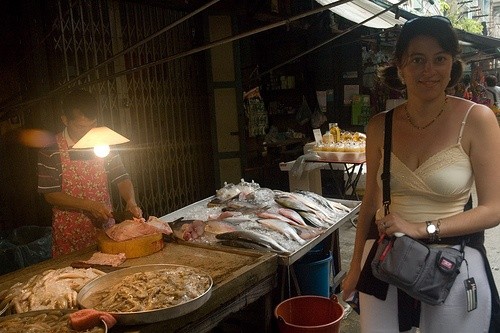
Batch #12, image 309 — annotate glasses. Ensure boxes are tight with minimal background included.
[399,15,451,31]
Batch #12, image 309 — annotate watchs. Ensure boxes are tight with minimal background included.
[425,220,437,242]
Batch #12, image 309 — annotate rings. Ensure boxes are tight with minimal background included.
[382,223,388,229]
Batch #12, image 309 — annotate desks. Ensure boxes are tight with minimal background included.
[303,157,367,202]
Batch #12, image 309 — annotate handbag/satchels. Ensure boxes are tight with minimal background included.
[370,231,465,306]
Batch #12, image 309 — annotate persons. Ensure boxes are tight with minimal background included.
[341,17,500,333]
[484,76,500,107]
[445,74,471,98]
[36,91,142,259]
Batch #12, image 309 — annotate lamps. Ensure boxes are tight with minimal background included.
[71,118,131,158]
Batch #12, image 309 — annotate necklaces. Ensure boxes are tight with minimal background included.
[405,94,449,130]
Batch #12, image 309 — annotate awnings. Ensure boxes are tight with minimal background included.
[46,0,500,95]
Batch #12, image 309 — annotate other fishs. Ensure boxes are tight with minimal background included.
[215,190,351,257]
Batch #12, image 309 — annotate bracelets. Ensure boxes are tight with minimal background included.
[436,218,441,240]
